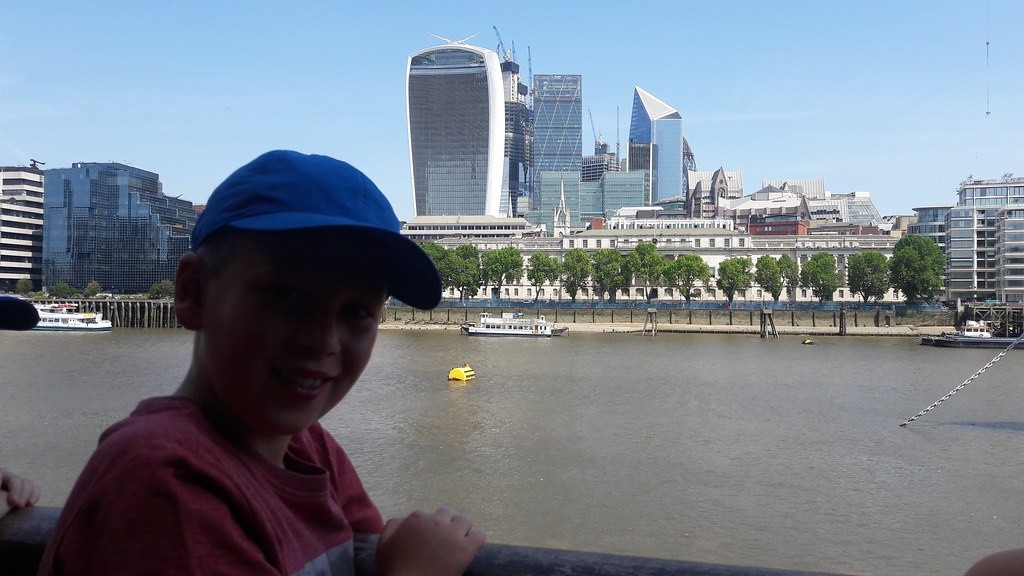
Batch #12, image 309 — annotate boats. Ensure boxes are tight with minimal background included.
[30,303,112,332]
[919,318,1024,349]
[460,312,569,338]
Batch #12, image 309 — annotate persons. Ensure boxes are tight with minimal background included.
[35,152,483,575]
[964,551,1024,576]
[0,467,40,518]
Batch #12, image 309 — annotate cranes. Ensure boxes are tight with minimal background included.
[527,45,533,111]
[510,40,518,65]
[588,109,603,155]
[493,25,513,63]
[615,106,620,170]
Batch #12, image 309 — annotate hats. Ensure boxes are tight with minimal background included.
[0,296,39,331]
[190,150,443,311]
